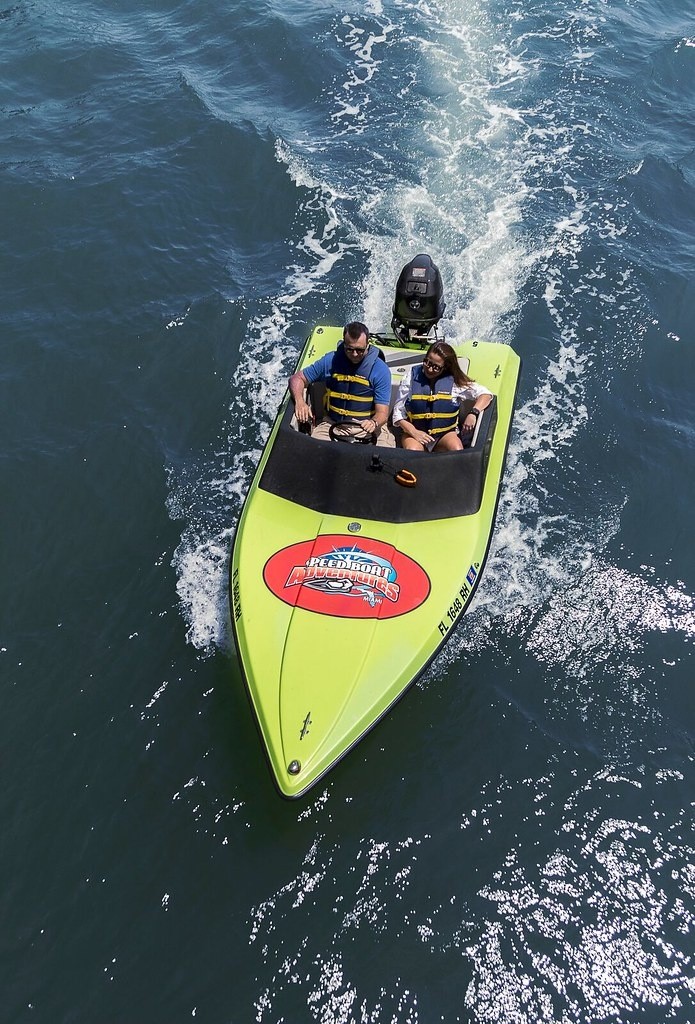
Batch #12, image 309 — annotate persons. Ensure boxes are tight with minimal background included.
[288,321,396,449]
[393,341,494,451]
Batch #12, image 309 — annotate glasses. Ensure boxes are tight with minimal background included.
[423,357,445,373]
[344,343,368,353]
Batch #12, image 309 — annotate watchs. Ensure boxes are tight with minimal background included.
[467,409,480,419]
[370,418,378,428]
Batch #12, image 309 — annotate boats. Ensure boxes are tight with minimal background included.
[228,254,522,802]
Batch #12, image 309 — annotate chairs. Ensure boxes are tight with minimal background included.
[457,397,476,446]
[309,380,328,428]
